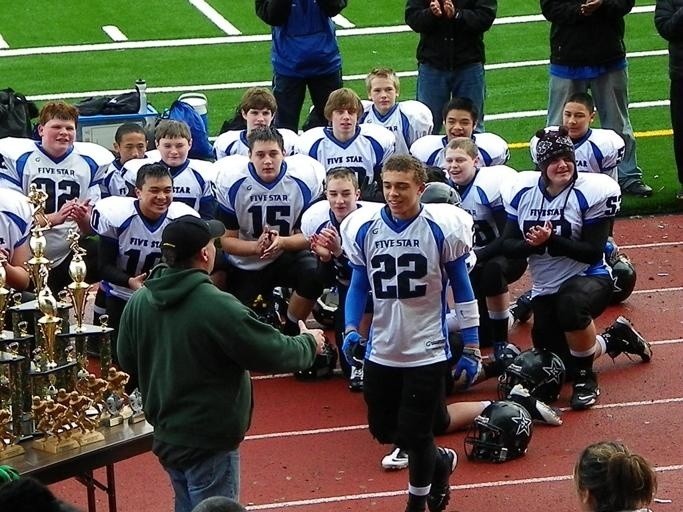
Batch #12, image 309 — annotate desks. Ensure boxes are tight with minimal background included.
[0,390,161,512]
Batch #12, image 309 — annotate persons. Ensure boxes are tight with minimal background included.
[573,440,656,512]
[340,151,482,512]
[647,0,682,185]
[117,216,325,512]
[254,0,357,138]
[1,71,652,470]
[536,3,651,197]
[403,1,501,136]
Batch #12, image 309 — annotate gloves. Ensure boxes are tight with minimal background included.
[342,330,369,369]
[453,347,482,387]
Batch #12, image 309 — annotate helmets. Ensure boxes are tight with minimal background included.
[462,401,532,463]
[498,349,564,404]
[312,288,341,327]
[421,182,463,208]
[607,253,636,302]
[295,336,339,378]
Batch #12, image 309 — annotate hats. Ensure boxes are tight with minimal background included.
[161,214,226,256]
[530,126,580,183]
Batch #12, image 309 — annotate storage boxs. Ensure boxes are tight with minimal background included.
[75,104,157,153]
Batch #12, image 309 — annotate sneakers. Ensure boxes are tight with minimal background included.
[382,448,409,469]
[426,448,457,512]
[627,183,653,198]
[508,383,562,425]
[518,291,533,323]
[494,344,522,367]
[606,315,652,362]
[272,299,300,335]
[569,373,599,410]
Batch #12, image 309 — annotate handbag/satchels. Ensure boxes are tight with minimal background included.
[170,100,213,161]
[75,93,140,115]
[1,88,38,137]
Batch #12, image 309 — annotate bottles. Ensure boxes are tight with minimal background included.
[134,78,147,114]
